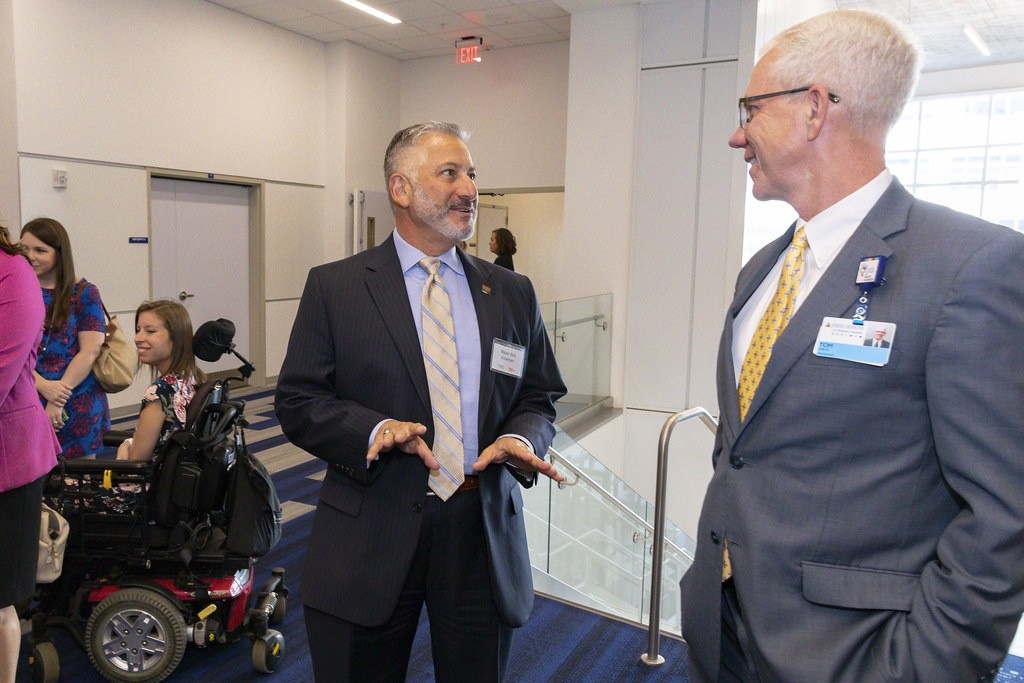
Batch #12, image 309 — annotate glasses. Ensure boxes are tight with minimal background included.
[739,85,839,130]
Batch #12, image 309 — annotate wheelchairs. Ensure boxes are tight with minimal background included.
[43,317,291,683]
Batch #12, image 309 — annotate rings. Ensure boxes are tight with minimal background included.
[53,421,58,424]
[384,429,389,434]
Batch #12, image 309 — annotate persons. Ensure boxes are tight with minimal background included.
[864,326,890,348]
[489,228,517,271]
[274,121,568,683]
[0,218,110,683]
[678,9,1024,683]
[115,300,208,459]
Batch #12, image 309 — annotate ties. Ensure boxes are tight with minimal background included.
[874,342,878,347]
[419,257,465,503]
[721,226,809,582]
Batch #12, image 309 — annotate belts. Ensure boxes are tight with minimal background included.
[427,475,479,495]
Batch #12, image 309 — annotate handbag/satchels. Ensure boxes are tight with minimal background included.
[77,282,141,393]
[36,502,69,582]
